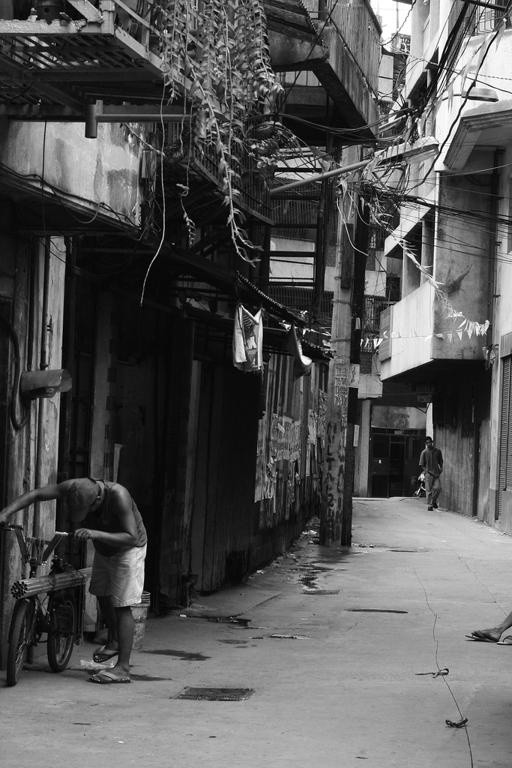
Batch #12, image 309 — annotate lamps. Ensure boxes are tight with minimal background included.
[264,134,439,194]
[379,88,499,132]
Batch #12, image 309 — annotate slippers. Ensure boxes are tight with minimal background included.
[90,644,131,683]
[464,630,512,645]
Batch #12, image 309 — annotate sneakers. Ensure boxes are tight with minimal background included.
[428,503,438,511]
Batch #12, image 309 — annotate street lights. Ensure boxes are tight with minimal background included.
[318,84,503,553]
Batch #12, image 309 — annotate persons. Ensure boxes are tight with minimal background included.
[0,478,148,684]
[419,437,443,511]
[464,611,512,645]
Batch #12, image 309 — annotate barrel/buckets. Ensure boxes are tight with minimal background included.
[129,590,151,649]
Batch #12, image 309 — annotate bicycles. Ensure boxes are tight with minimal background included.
[0,518,86,685]
